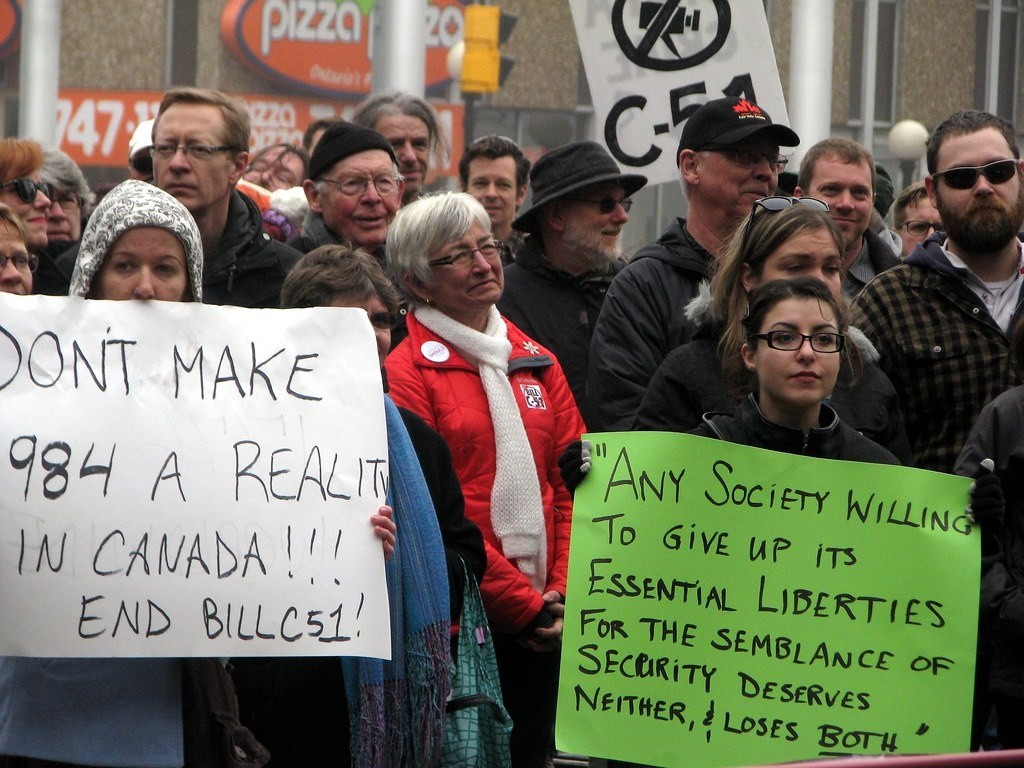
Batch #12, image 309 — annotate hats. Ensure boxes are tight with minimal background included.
[510,141,648,233]
[676,96,800,162]
[308,120,400,183]
[128,118,155,158]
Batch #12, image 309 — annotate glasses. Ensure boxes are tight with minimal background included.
[46,194,81,209]
[316,175,396,196]
[899,218,944,236]
[558,196,634,213]
[425,238,503,268]
[148,144,234,162]
[758,330,846,353]
[739,197,831,241]
[368,309,399,329]
[129,156,153,173]
[0,177,49,205]
[695,146,788,173]
[929,158,1016,190]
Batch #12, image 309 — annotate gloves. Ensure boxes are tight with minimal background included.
[967,457,1005,527]
[556,440,591,496]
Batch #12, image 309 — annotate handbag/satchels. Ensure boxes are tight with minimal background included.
[442,554,515,768]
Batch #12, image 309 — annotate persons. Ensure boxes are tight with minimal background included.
[0,87,1024,768]
